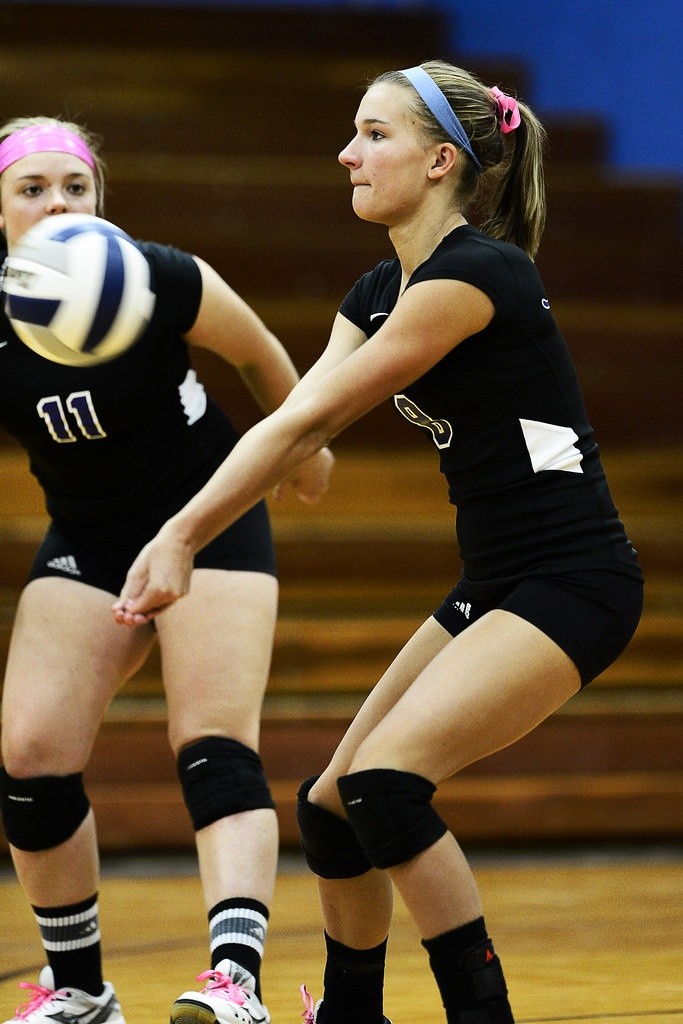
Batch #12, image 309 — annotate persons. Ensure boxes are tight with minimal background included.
[111,58,646,1024]
[0,110,340,1024]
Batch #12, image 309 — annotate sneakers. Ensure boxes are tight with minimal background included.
[169,958,271,1024]
[0,965,127,1024]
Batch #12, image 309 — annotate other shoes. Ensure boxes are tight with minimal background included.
[300,984,394,1024]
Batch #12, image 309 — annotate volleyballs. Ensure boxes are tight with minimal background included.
[2,209,160,369]
